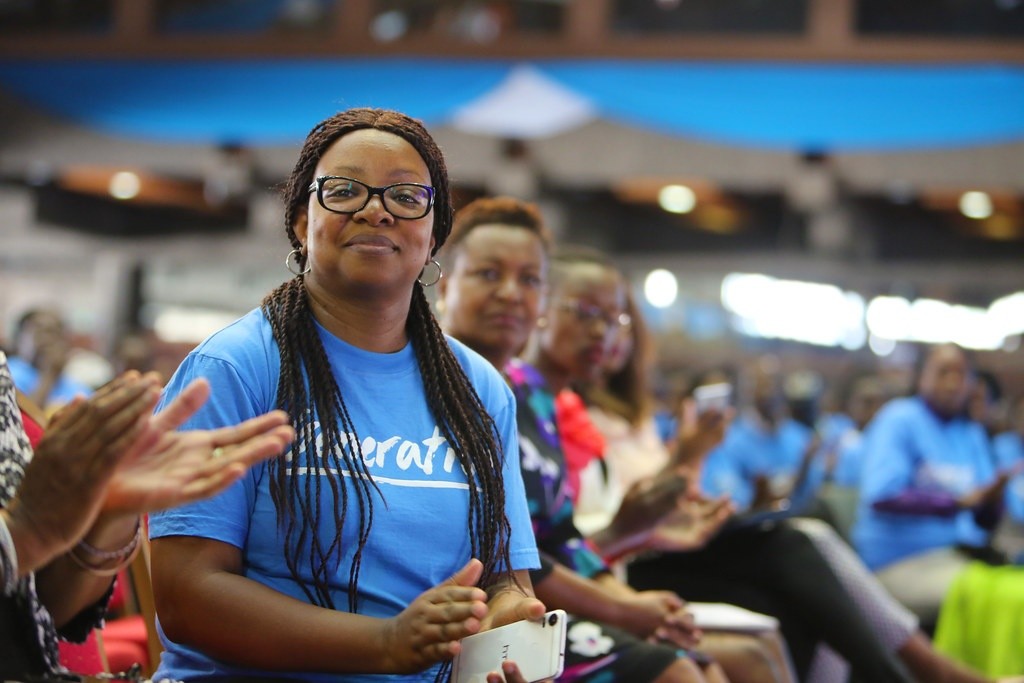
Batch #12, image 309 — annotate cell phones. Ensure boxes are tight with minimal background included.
[450,609,568,683]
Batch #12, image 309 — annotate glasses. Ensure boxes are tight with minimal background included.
[548,297,631,330]
[308,175,436,220]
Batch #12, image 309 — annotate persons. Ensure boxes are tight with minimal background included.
[147,108,564,683]
[524,250,1024,683]
[1,310,295,683]
[435,197,733,681]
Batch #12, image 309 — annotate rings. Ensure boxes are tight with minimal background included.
[211,446,223,457]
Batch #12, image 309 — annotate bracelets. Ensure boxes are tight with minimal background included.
[70,516,143,577]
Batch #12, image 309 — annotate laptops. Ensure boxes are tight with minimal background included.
[731,433,836,527]
